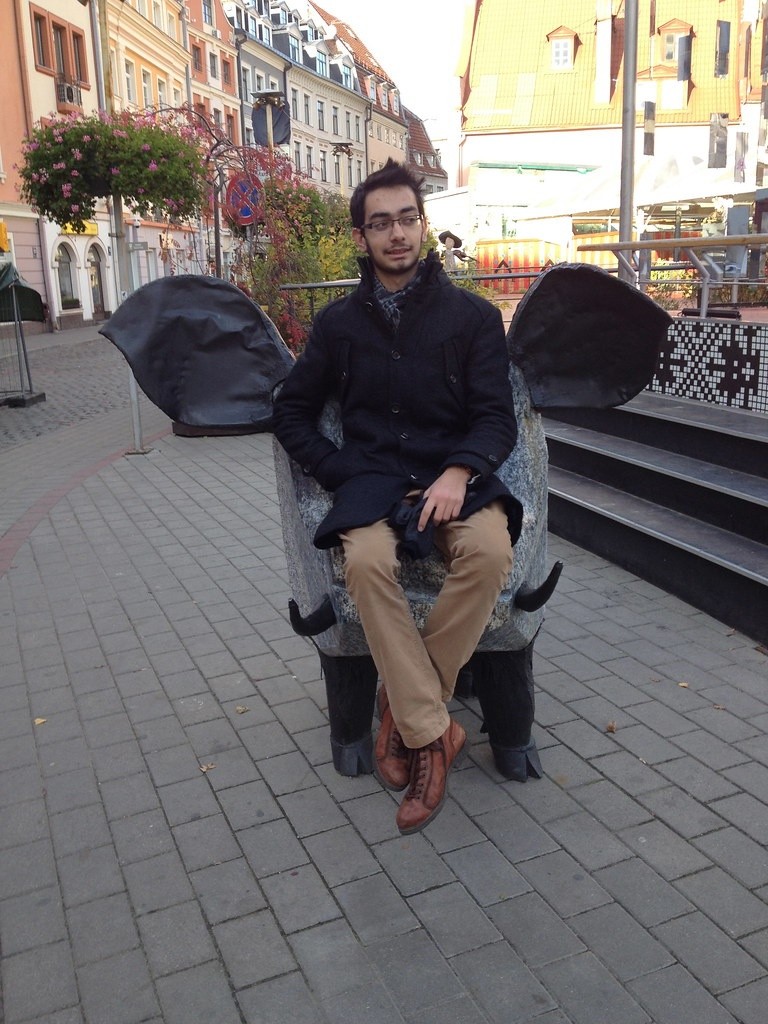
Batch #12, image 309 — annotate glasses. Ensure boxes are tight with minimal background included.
[357,214,424,232]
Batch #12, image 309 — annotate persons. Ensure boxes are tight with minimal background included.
[271,156,526,834]
[437,230,473,276]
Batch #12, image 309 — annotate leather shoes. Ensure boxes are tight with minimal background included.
[375,685,410,793]
[394,720,470,836]
